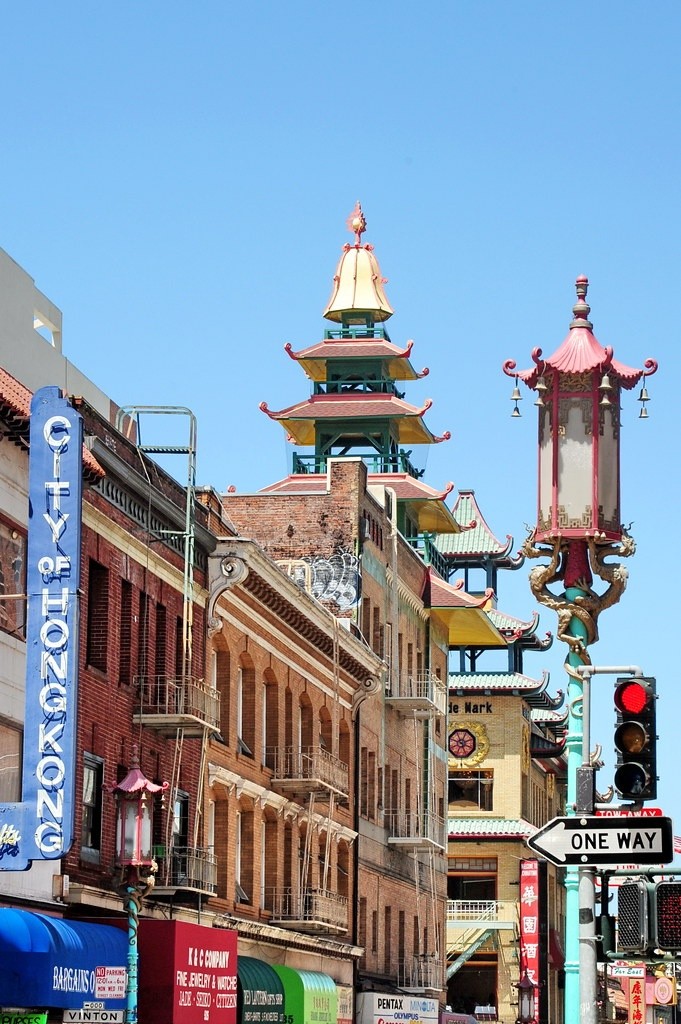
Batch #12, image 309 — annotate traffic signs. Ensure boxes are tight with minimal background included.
[525,817,675,867]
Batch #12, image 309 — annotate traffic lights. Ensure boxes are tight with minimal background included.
[616,883,647,951]
[614,676,659,801]
[656,880,681,951]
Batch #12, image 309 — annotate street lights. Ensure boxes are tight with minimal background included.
[502,276,658,1024]
